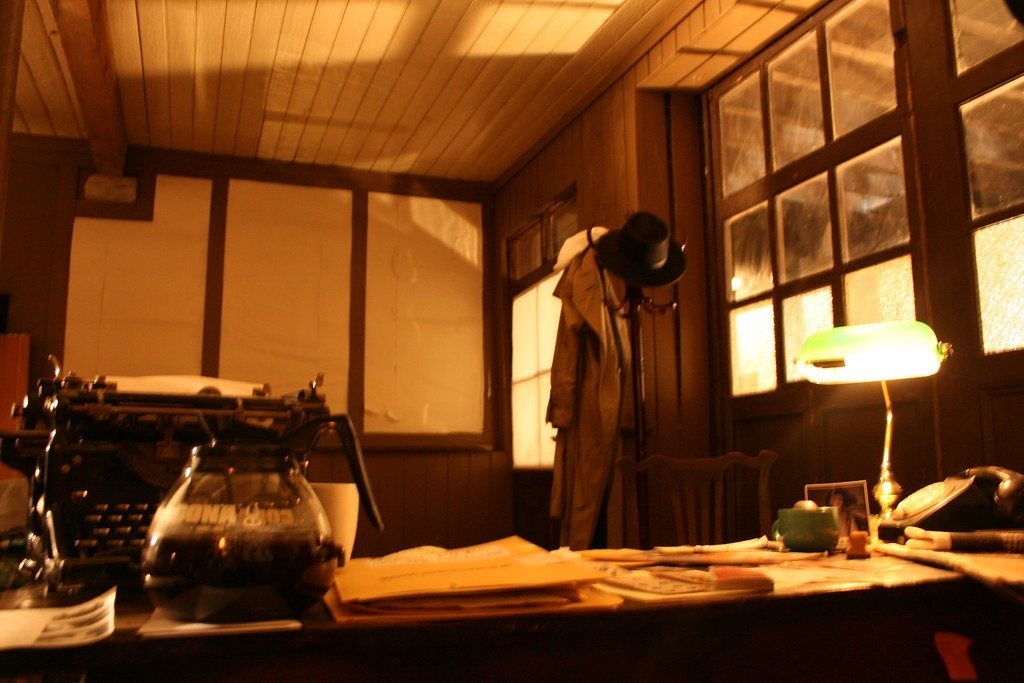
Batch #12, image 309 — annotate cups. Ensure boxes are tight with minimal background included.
[772,509,841,553]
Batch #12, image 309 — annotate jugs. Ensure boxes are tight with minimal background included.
[142,412,385,623]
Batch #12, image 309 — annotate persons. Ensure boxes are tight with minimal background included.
[828,491,857,538]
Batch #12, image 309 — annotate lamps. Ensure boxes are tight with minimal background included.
[794,322,954,516]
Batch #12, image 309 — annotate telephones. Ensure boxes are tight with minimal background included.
[878,464,1024,544]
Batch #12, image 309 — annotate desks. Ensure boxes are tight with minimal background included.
[0,549,967,683]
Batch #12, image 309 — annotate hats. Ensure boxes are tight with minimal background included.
[597,212,687,287]
